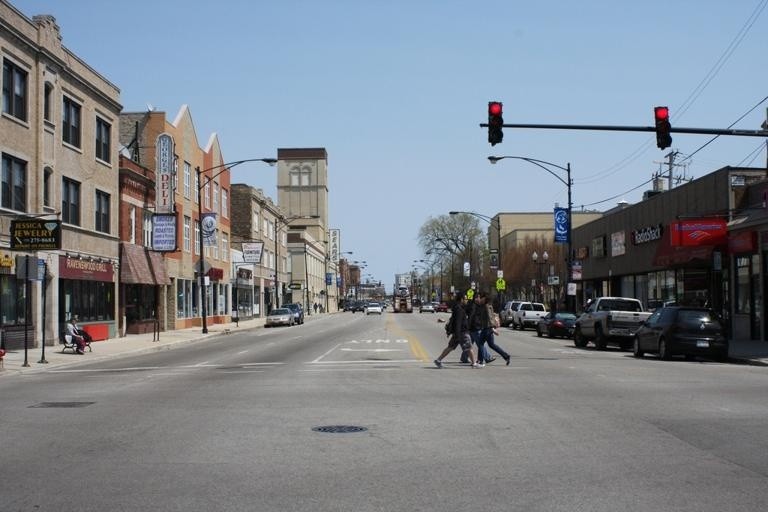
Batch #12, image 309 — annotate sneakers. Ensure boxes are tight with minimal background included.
[505,355,511,365]
[460,358,496,368]
[433,360,444,369]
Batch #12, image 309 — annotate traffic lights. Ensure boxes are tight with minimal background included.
[654,106,672,149]
[488,102,504,144]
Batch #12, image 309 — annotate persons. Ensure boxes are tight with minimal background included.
[318,303,323,312]
[66,318,86,354]
[314,302,319,313]
[434,291,511,368]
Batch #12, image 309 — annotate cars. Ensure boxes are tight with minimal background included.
[267,308,295,327]
[281,303,304,325]
[633,305,728,361]
[537,312,577,340]
[397,286,410,297]
[341,299,390,315]
[420,301,448,313]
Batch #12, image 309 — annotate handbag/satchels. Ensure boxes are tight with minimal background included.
[444,317,453,335]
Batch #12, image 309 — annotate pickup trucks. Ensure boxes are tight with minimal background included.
[573,296,654,351]
[500,301,551,331]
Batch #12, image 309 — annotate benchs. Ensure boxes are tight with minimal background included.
[61,330,92,355]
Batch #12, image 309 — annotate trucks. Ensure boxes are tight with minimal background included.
[393,289,413,313]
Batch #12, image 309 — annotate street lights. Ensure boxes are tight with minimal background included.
[488,155,574,314]
[345,260,376,300]
[196,159,279,334]
[533,251,549,302]
[412,238,472,303]
[324,251,352,313]
[449,212,502,315]
[274,215,321,310]
